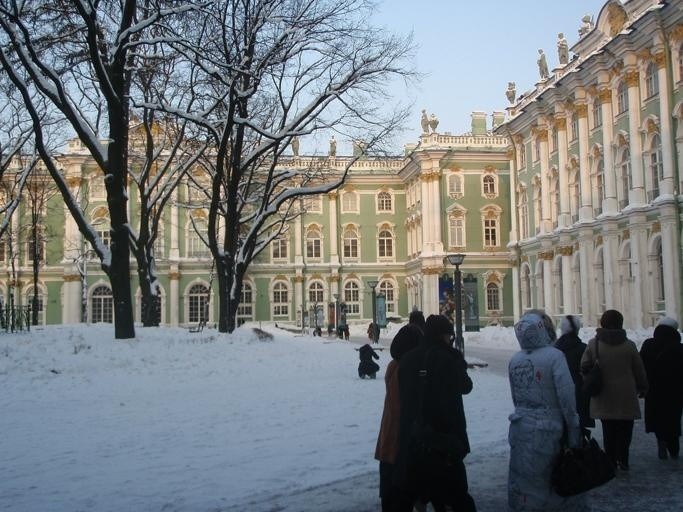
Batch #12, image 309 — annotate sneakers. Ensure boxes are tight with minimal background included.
[658,443,680,460]
[618,459,629,470]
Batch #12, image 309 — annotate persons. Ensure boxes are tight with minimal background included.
[371,312,477,512]
[553,310,683,470]
[506,309,583,511]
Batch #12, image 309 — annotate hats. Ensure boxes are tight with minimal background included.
[660,316,678,330]
[391,314,455,357]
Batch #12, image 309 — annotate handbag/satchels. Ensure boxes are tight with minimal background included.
[576,367,603,394]
[554,440,616,497]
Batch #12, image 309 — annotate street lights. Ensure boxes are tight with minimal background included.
[367,280,380,344]
[333,294,340,337]
[447,254,466,358]
